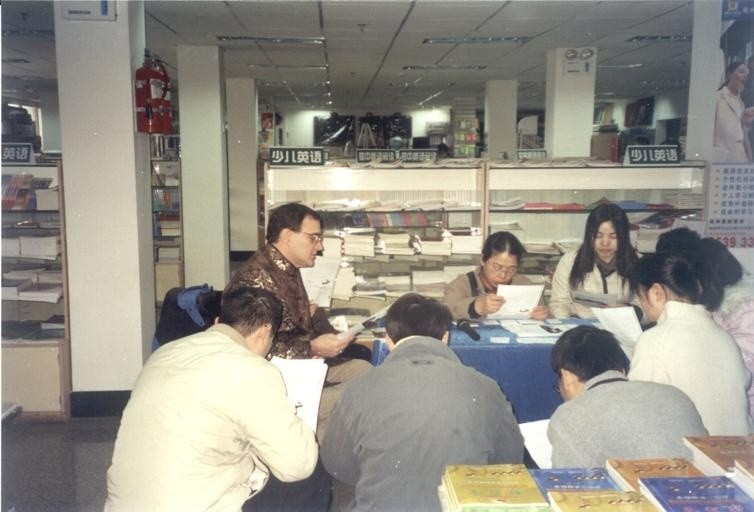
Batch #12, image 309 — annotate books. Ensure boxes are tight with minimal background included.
[152,169,183,305]
[1,171,66,346]
[297,199,483,338]
[489,191,703,294]
[434,436,754,512]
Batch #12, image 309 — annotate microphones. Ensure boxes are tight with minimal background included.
[457,318,481,341]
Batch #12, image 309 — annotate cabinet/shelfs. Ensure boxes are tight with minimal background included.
[264,160,710,326]
[427,119,685,159]
[2,162,71,422]
[148,158,183,308]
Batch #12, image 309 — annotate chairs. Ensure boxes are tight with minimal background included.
[148,285,223,353]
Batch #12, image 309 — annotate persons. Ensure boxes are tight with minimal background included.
[550,203,655,328]
[217,202,373,446]
[102,288,332,511]
[442,230,549,321]
[317,291,526,511]
[656,224,754,418]
[545,324,710,467]
[627,249,754,436]
[713,60,753,164]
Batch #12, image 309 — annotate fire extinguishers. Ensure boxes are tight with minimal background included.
[135,48,162,134]
[154,56,171,135]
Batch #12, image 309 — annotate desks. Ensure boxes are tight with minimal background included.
[371,318,628,466]
